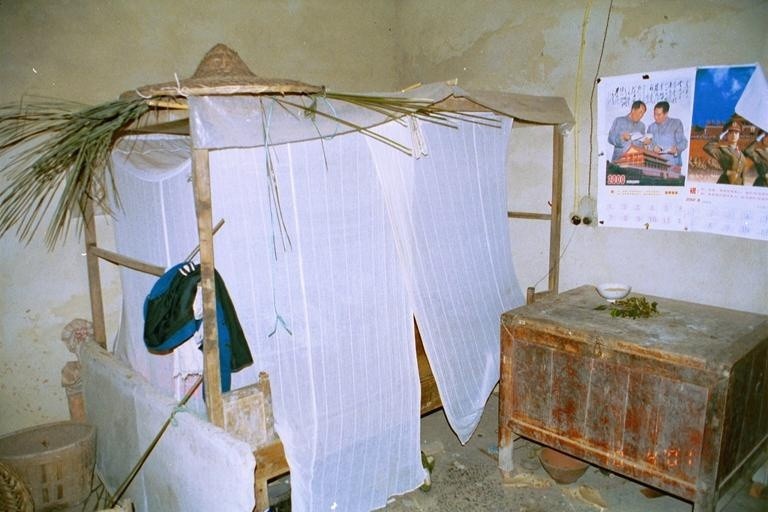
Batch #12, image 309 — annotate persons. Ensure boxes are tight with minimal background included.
[608,100,646,161]
[703,120,746,185]
[647,101,688,174]
[742,129,768,186]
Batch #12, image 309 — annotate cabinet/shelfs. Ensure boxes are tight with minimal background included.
[496,282,767,512]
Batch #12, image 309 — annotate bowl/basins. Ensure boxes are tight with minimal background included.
[538,446,589,484]
[597,282,631,304]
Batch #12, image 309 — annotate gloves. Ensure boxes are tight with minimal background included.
[756,133,766,142]
[720,130,728,140]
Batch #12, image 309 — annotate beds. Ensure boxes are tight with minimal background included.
[78,91,562,512]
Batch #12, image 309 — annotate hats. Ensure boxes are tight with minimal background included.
[723,121,742,132]
[120,44,323,95]
